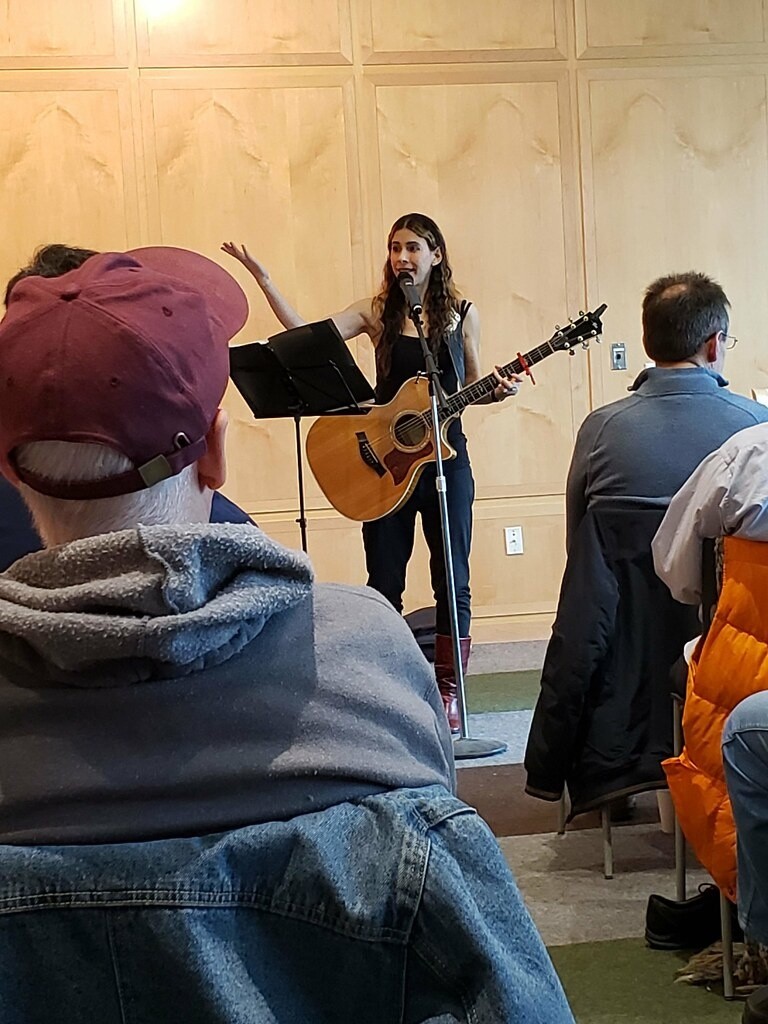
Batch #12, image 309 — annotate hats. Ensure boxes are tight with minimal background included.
[0,246,249,501]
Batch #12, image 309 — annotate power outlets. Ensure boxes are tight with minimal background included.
[504,526,524,555]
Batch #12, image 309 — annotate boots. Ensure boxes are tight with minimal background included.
[434,633,472,734]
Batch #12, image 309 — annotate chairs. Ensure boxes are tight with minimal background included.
[0,494,767,1024]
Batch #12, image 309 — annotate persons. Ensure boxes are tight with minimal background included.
[0,244,456,1024]
[523,270,768,1024]
[221,213,523,734]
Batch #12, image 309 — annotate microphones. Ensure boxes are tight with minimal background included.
[397,272,423,313]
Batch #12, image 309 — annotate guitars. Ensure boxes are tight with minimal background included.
[306,302,608,524]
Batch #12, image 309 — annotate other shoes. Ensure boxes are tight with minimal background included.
[643,881,743,947]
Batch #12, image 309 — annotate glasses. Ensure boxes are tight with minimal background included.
[704,329,737,350]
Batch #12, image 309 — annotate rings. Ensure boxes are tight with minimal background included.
[503,389,508,393]
[510,387,517,391]
